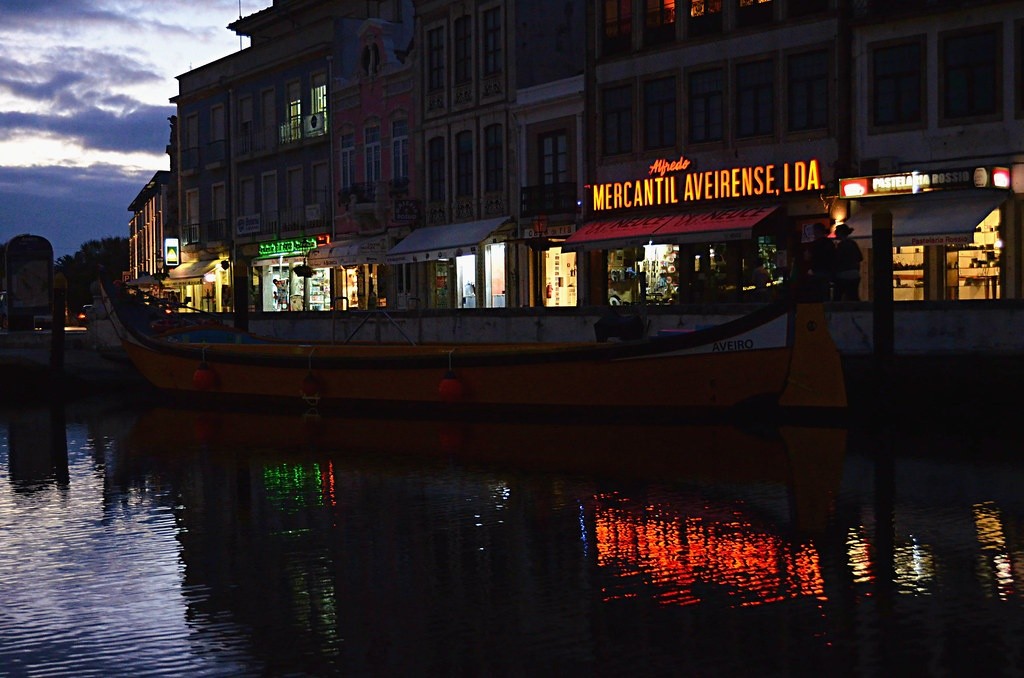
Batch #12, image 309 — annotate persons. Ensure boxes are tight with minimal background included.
[131,287,182,314]
[834,225,863,302]
[810,222,834,304]
[684,250,778,305]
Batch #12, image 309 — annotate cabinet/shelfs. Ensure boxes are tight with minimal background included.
[310,269,325,311]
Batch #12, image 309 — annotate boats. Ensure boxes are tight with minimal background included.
[97,267,851,423]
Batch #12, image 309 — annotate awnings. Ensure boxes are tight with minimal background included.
[385,216,510,265]
[160,259,226,286]
[560,204,782,253]
[834,189,1010,244]
[307,235,391,268]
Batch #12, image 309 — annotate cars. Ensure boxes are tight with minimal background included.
[76,304,96,324]
[0,291,8,329]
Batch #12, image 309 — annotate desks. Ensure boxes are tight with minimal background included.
[896,267,1000,300]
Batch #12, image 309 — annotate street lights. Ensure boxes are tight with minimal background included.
[155,255,165,299]
